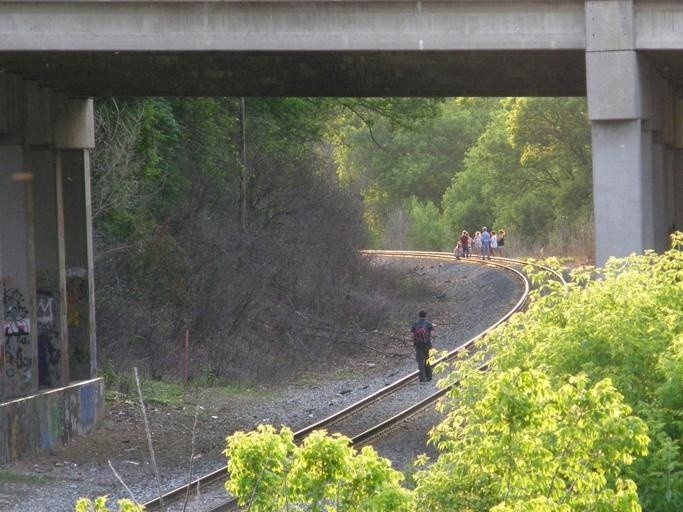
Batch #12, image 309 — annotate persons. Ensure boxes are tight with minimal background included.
[453,226,505,261]
[408,310,434,382]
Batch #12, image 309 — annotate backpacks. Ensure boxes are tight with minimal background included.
[416,321,427,344]
